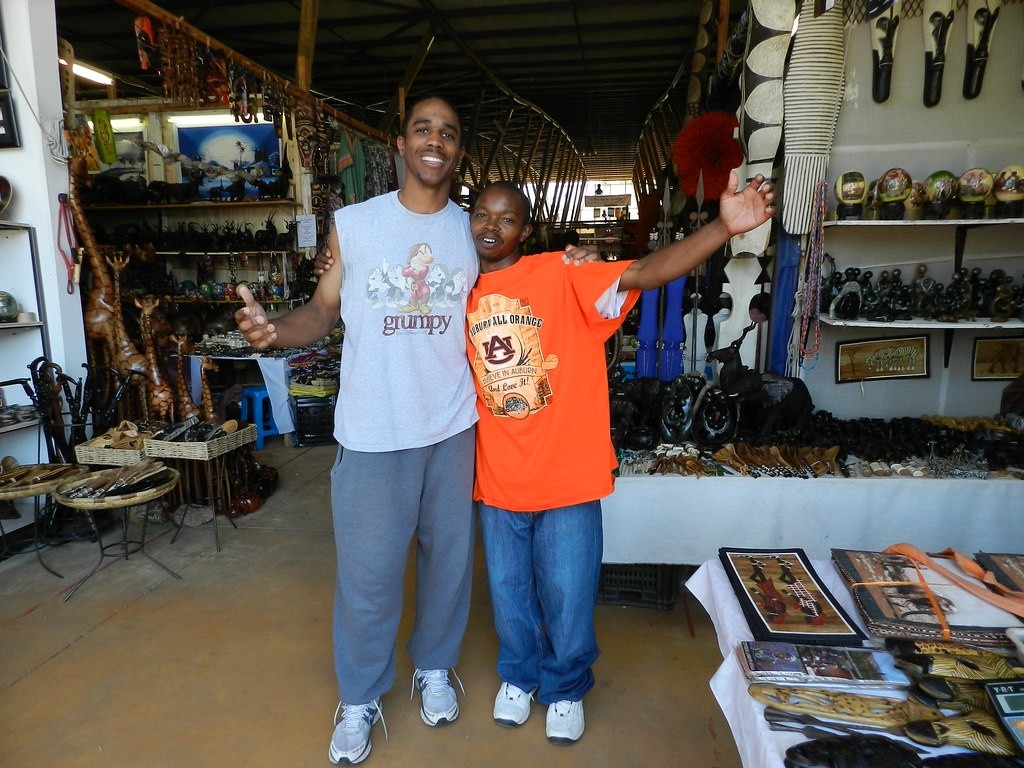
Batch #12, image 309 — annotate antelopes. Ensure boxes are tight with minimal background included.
[710,321,816,447]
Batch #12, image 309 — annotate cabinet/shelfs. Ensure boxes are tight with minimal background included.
[69,105,318,355]
[0,218,76,546]
[814,164,1024,338]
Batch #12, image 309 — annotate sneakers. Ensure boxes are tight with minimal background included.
[546,698,585,743]
[329,697,385,763]
[414,666,461,726]
[492,682,538,725]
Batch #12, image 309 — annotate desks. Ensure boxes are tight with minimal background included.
[596,419,1023,575]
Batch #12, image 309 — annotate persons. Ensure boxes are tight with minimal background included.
[310,168,776,748]
[233,81,607,765]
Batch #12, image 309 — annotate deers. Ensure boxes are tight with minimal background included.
[194,355,226,430]
[169,332,202,422]
[134,297,175,425]
[69,147,117,424]
[105,250,150,425]
